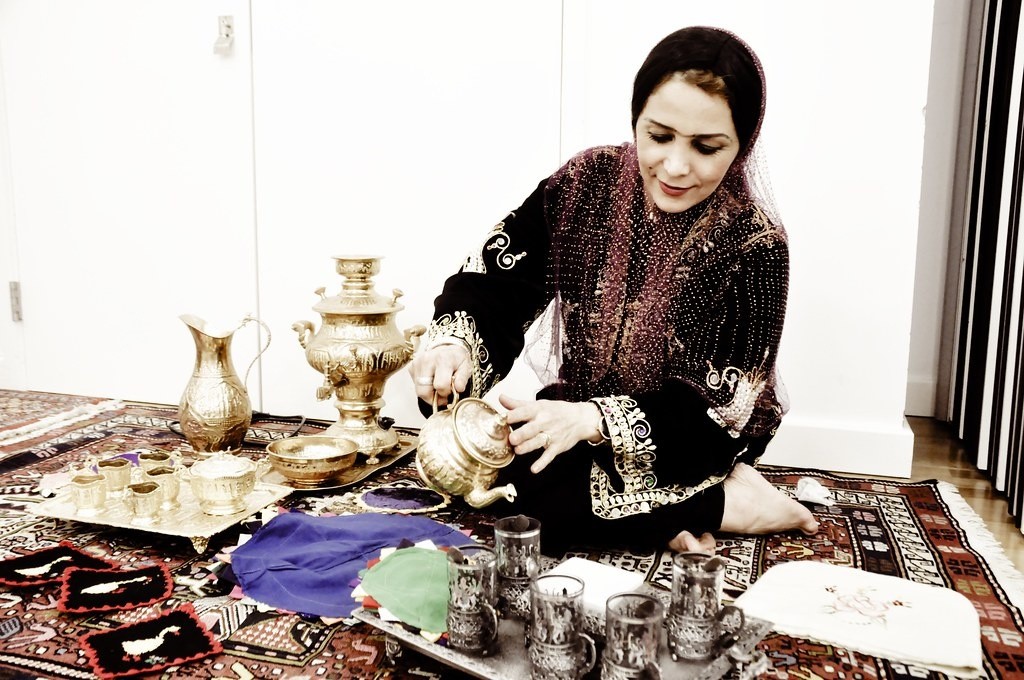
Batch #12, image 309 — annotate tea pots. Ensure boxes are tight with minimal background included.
[415,377,517,508]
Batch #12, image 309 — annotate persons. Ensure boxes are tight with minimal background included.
[412,25,817,558]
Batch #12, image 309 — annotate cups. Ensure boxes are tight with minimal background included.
[145,466,180,511]
[667,551,744,660]
[97,460,132,494]
[600,593,665,680]
[138,451,170,476]
[446,543,498,659]
[495,517,540,618]
[528,575,596,680]
[127,481,162,525]
[71,475,108,517]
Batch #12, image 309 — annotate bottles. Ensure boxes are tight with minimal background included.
[292,255,427,464]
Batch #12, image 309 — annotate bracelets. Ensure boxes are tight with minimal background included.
[589,400,611,446]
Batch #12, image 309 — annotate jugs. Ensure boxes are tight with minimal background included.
[178,313,271,452]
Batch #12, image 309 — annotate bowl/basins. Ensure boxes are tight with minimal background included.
[181,450,258,516]
[266,438,359,484]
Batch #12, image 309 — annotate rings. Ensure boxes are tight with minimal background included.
[542,432,551,445]
[416,376,433,385]
